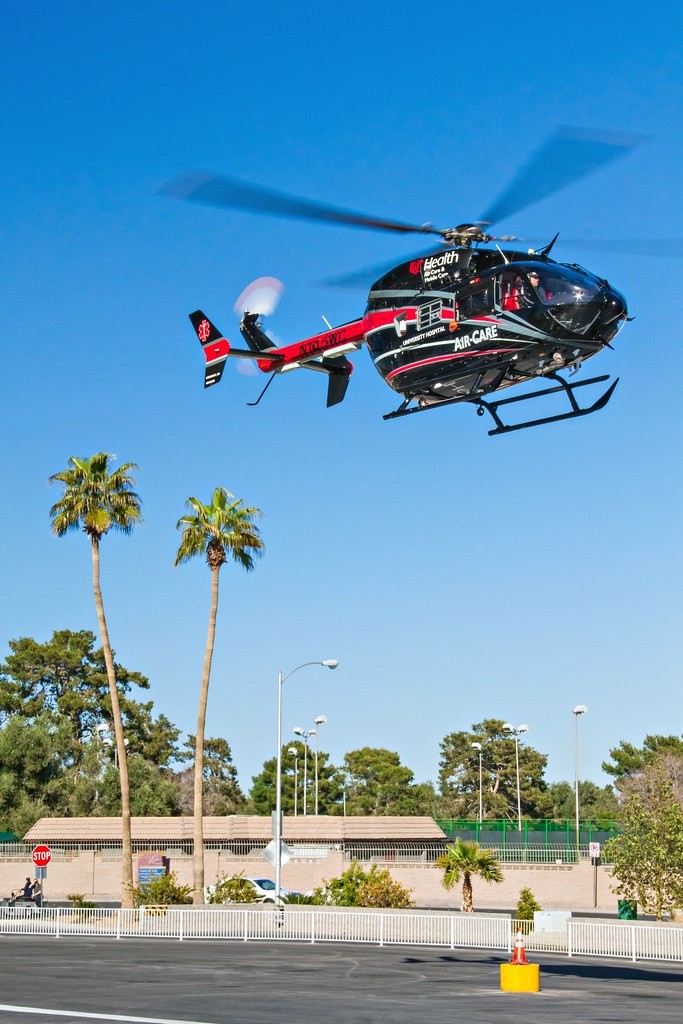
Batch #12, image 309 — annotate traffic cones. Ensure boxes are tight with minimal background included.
[509,927,529,965]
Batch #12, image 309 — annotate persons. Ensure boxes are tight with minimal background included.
[20,877,33,897]
[518,272,547,308]
[29,879,41,903]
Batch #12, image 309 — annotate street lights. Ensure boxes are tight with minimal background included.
[293,726,316,815]
[273,657,338,928]
[312,714,329,816]
[573,705,587,860]
[471,741,483,831]
[288,747,299,817]
[502,723,529,831]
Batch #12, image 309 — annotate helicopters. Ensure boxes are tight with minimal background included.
[157,125,682,438]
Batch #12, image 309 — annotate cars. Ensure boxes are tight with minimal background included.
[204,875,303,904]
[305,877,379,907]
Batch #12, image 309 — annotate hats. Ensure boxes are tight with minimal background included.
[528,270,542,276]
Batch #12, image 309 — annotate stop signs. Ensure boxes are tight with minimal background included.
[31,844,52,867]
